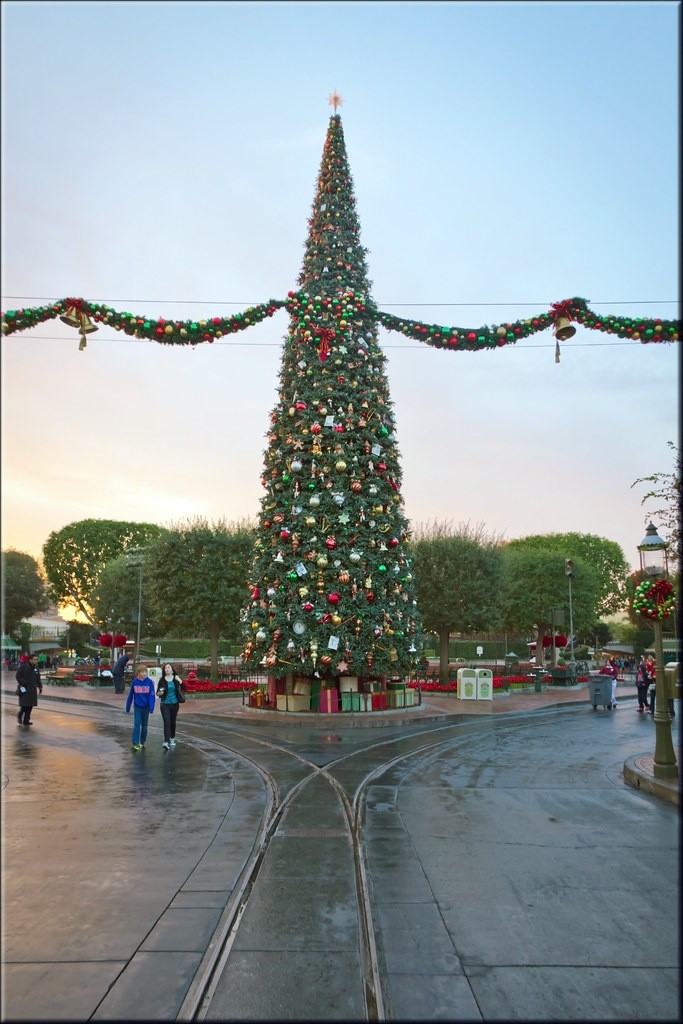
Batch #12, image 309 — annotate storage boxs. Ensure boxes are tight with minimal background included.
[275,683,416,713]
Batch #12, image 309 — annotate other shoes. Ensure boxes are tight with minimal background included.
[162,742,170,750]
[130,746,139,752]
[23,720,33,725]
[637,706,644,711]
[169,738,175,746]
[140,744,146,749]
[645,706,649,711]
[613,705,616,709]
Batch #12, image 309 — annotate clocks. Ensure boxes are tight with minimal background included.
[293,620,307,634]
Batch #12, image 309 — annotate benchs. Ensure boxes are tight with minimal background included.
[411,665,536,683]
[196,664,249,684]
[45,667,77,687]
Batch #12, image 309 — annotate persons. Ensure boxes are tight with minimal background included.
[16,655,43,724]
[611,654,636,674]
[592,659,618,709]
[637,655,656,714]
[156,664,186,750]
[38,651,45,668]
[95,651,100,666]
[112,652,133,694]
[126,666,155,751]
[19,651,29,664]
[4,658,9,672]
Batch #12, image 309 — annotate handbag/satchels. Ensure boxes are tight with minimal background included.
[15,685,25,696]
[178,685,185,703]
[18,712,22,724]
